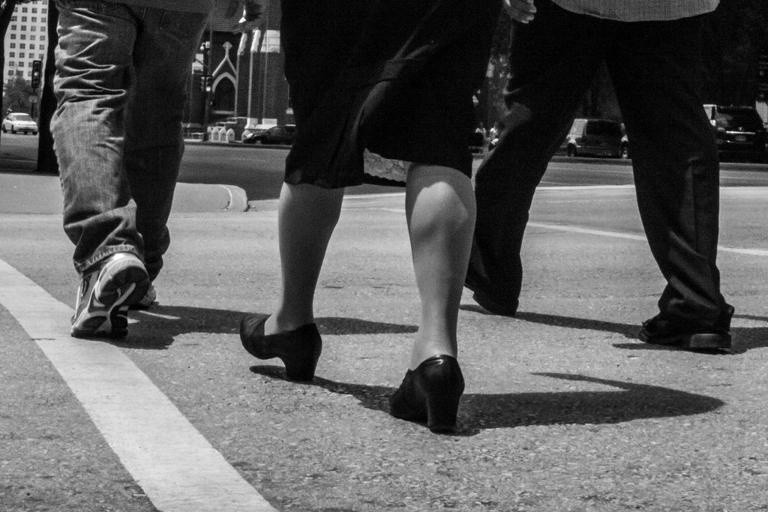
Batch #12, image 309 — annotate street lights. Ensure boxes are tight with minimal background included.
[199,41,212,143]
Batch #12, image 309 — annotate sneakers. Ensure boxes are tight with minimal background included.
[71,252,155,337]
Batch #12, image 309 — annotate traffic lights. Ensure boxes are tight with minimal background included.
[199,77,204,92]
[31,60,42,89]
[205,77,212,93]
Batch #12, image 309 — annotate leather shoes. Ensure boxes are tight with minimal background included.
[639,311,732,349]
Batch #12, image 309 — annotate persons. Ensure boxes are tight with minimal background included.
[464,0,735,350]
[238,0,504,431]
[49,0,267,340]
[566,131,579,158]
[489,120,502,142]
[475,121,487,145]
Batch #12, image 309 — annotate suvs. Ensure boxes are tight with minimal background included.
[556,116,623,158]
[701,102,765,163]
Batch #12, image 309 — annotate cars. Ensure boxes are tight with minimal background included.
[1,111,38,135]
[620,134,630,159]
[241,122,296,146]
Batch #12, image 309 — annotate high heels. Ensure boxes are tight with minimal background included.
[241,314,321,380]
[389,355,464,431]
